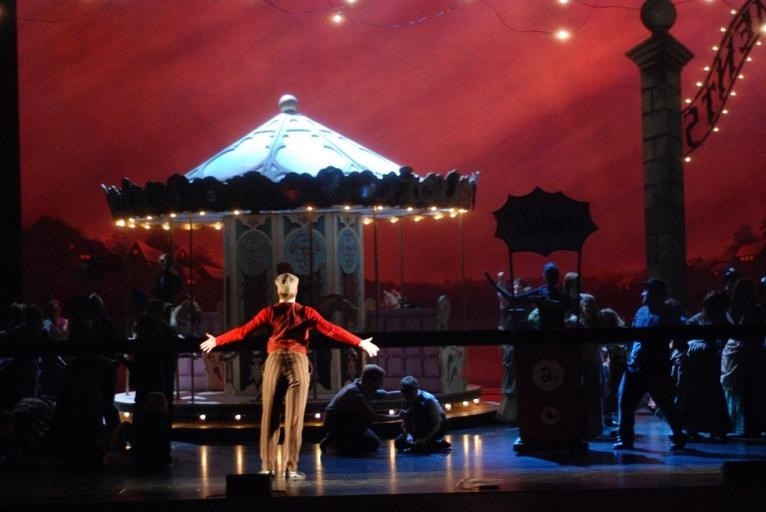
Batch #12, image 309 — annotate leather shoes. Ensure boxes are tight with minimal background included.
[256,470,307,480]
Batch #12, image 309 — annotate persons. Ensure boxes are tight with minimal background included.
[199,262,380,479]
[2,253,199,480]
[485,263,764,451]
[395,376,452,451]
[320,363,408,458]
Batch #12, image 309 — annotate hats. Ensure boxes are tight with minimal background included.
[274,273,300,294]
